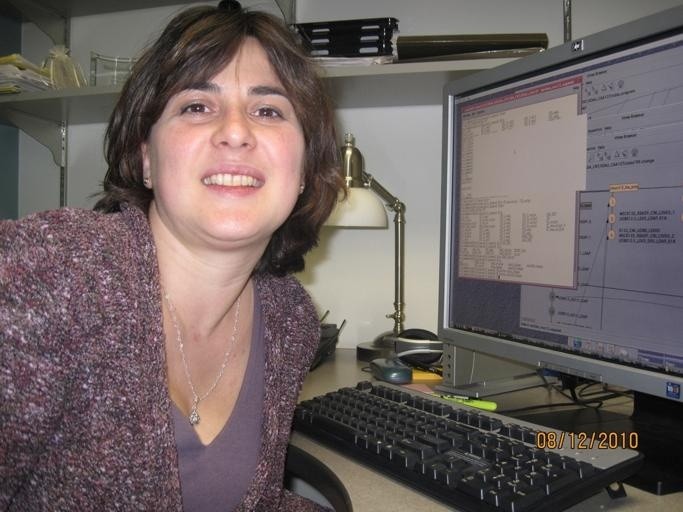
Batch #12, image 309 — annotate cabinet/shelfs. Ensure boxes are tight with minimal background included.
[1,0,576,129]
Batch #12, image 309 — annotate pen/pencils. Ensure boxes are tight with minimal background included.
[438,394,498,412]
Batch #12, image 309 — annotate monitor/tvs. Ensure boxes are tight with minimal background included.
[436,5,683,496]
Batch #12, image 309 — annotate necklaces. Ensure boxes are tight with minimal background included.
[157,288,241,428]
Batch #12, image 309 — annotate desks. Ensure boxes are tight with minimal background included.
[289,346,682,511]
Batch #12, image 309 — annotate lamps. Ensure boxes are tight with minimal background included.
[327,130,430,366]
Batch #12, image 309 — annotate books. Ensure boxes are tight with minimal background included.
[396,33,548,62]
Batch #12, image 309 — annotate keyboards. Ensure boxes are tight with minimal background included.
[289,378,644,512]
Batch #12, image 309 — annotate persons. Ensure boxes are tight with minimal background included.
[0,0,344,512]
